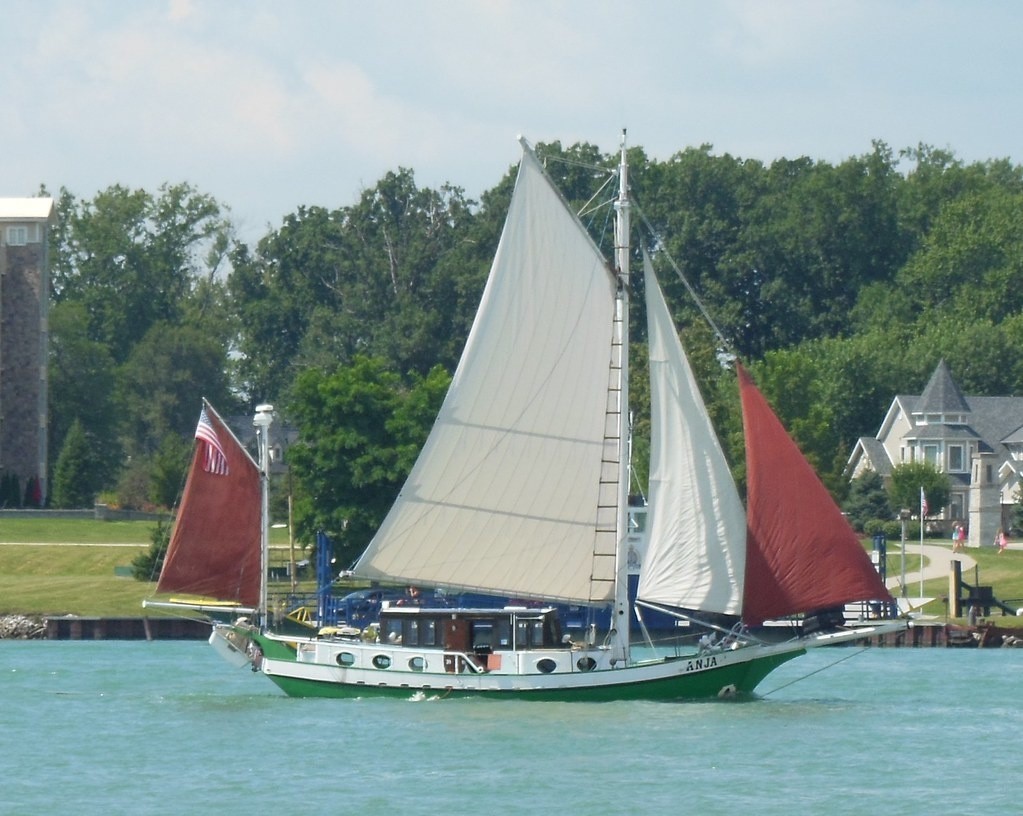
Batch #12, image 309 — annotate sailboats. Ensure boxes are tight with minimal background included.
[142,125,914,703]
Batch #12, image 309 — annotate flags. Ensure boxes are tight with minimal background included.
[195,410,229,476]
[922,493,928,518]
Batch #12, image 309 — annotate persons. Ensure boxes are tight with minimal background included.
[396,585,421,606]
[951,521,969,554]
[994,526,1007,556]
